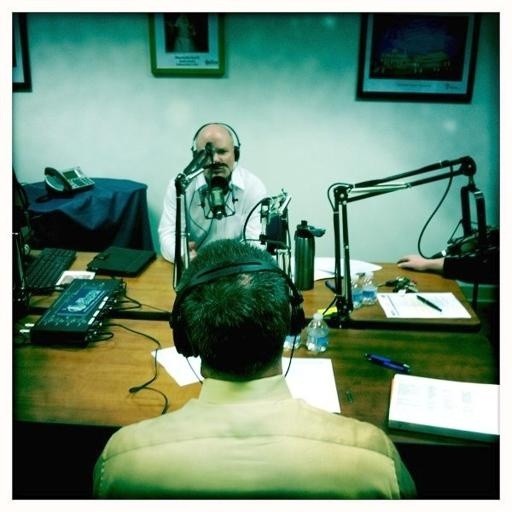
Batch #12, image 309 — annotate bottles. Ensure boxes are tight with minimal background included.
[364,272,377,306]
[306,312,330,353]
[351,275,363,309]
[282,334,301,351]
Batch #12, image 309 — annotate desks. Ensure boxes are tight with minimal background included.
[14,250,499,499]
[21,176,148,253]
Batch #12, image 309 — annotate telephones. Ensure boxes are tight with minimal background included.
[45,166,95,193]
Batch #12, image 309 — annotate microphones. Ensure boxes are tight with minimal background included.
[211,175,230,221]
[432,223,500,261]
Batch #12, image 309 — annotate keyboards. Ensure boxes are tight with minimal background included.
[25,247,77,289]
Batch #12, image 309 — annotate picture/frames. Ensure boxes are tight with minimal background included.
[12,12,33,92]
[147,13,225,77]
[355,12,481,104]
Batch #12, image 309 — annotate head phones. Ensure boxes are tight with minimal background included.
[192,122,241,162]
[169,257,306,359]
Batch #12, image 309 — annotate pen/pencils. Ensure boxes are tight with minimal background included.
[417,296,442,312]
[364,353,410,371]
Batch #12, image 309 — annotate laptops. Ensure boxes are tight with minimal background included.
[86,244,157,278]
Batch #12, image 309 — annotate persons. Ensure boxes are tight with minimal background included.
[90,239,419,500]
[395,245,500,284]
[157,122,272,259]
